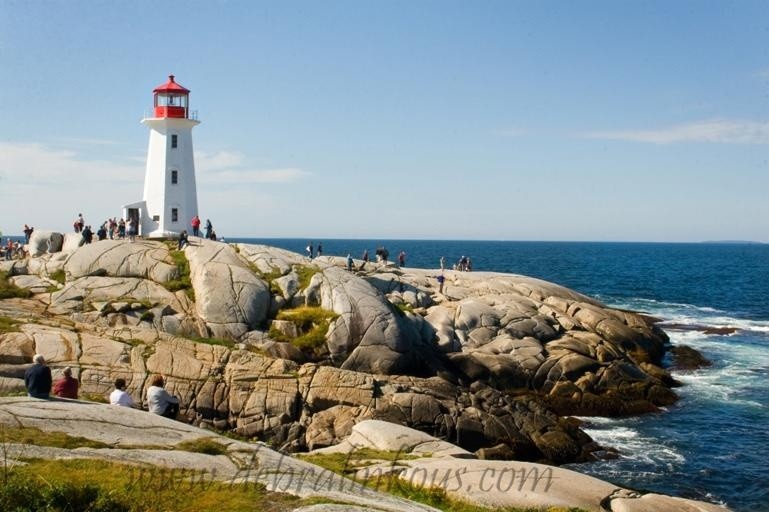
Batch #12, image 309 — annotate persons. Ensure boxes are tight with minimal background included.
[440,255,473,272]
[191,215,227,243]
[345,243,407,273]
[108,377,135,408]
[316,241,322,257]
[23,353,53,399]
[306,241,313,261]
[177,230,191,251]
[437,273,446,294]
[145,373,182,419]
[53,366,78,400]
[0,221,35,261]
[71,211,138,247]
